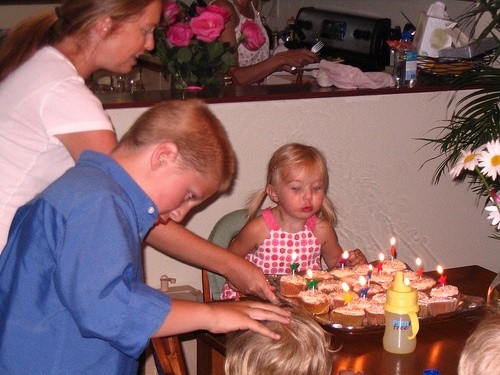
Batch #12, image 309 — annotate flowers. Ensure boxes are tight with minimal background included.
[156,0,266,90]
[449,140,500,236]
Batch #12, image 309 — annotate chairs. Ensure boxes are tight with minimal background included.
[201,209,262,302]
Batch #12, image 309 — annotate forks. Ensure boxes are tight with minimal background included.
[291,40,325,71]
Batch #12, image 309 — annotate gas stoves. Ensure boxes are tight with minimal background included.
[285,6,392,72]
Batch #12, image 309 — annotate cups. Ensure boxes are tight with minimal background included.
[110,72,131,93]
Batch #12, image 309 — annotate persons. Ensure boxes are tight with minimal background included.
[0,99,291,375]
[458,314,500,375]
[225,302,342,375]
[220,142,368,301]
[206,0,318,85]
[0,0,282,305]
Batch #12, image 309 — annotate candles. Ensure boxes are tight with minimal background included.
[291,237,446,309]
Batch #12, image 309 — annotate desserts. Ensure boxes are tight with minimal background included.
[279,258,460,326]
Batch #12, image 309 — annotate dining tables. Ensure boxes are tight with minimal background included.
[194,264,500,375]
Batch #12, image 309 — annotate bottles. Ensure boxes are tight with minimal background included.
[382,272,420,356]
[286,16,296,42]
[393,32,418,89]
